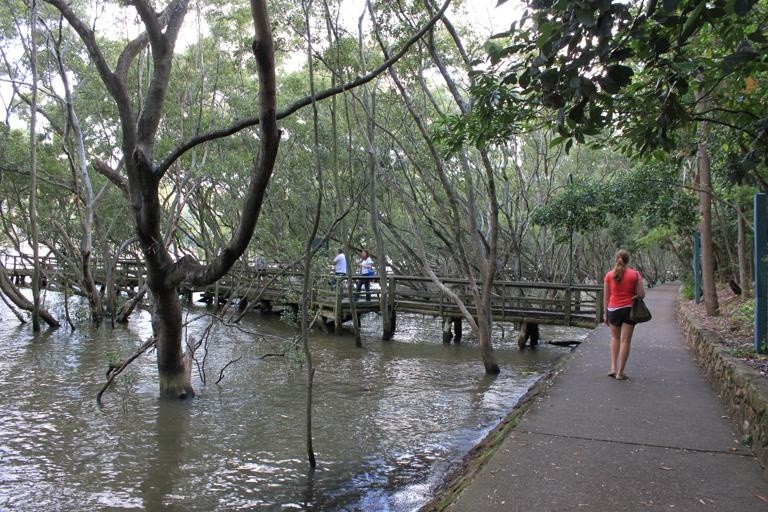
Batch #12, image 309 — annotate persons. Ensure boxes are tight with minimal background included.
[353,250,374,303]
[601,248,646,380]
[254,249,267,279]
[329,248,346,300]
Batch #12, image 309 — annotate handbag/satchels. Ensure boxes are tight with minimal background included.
[629,270,652,323]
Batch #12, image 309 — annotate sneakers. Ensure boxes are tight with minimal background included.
[607,368,629,381]
